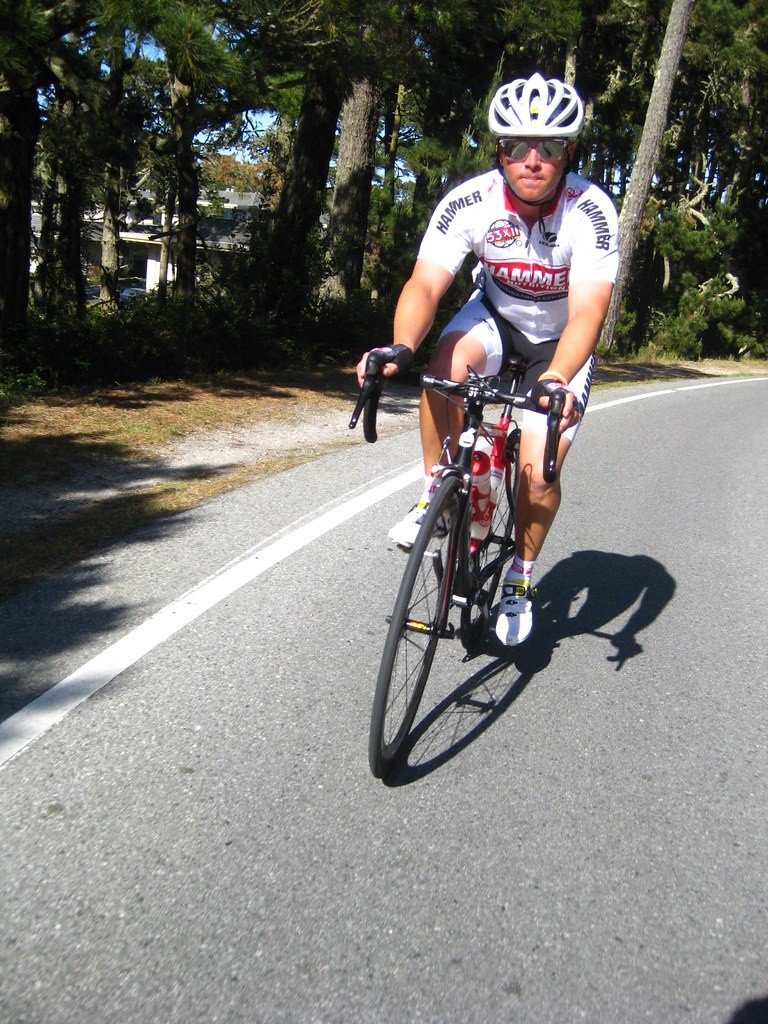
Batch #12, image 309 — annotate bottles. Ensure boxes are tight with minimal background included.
[490,455,505,507]
[469,451,493,540]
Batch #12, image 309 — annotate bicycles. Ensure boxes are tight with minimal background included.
[346,351,565,777]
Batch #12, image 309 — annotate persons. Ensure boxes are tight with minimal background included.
[357,72,622,647]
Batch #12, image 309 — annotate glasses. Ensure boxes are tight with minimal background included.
[498,138,573,162]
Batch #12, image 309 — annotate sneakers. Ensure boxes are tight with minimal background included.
[388,501,450,548]
[496,577,532,646]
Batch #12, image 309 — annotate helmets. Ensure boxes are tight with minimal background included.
[488,71,584,138]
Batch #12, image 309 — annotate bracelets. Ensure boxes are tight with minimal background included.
[538,370,567,386]
